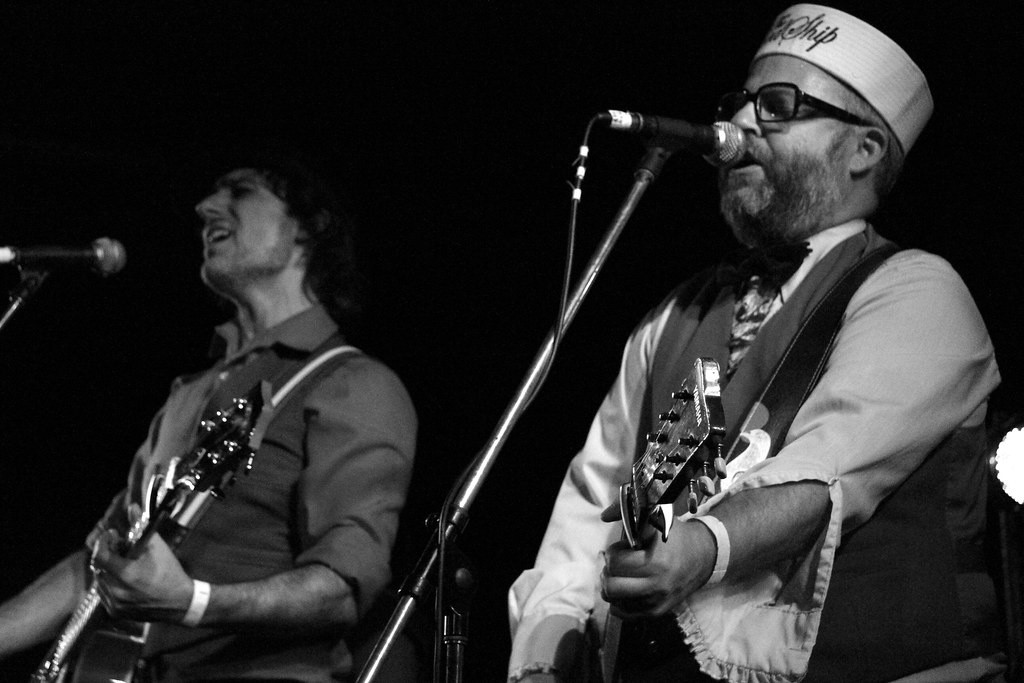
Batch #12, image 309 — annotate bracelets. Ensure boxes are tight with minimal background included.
[180,579,213,630]
[687,513,731,588]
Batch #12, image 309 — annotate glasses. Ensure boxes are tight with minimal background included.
[715,81,878,129]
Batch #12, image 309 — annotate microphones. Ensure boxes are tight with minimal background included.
[595,110,749,167]
[0,237,125,276]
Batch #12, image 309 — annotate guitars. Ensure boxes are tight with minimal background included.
[534,356,730,672]
[19,376,270,683]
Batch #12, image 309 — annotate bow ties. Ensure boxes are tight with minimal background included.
[735,243,804,285]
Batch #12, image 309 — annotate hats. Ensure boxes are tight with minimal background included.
[749,3,935,159]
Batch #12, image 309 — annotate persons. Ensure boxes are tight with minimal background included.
[0,153,422,683]
[504,2,1010,681]
